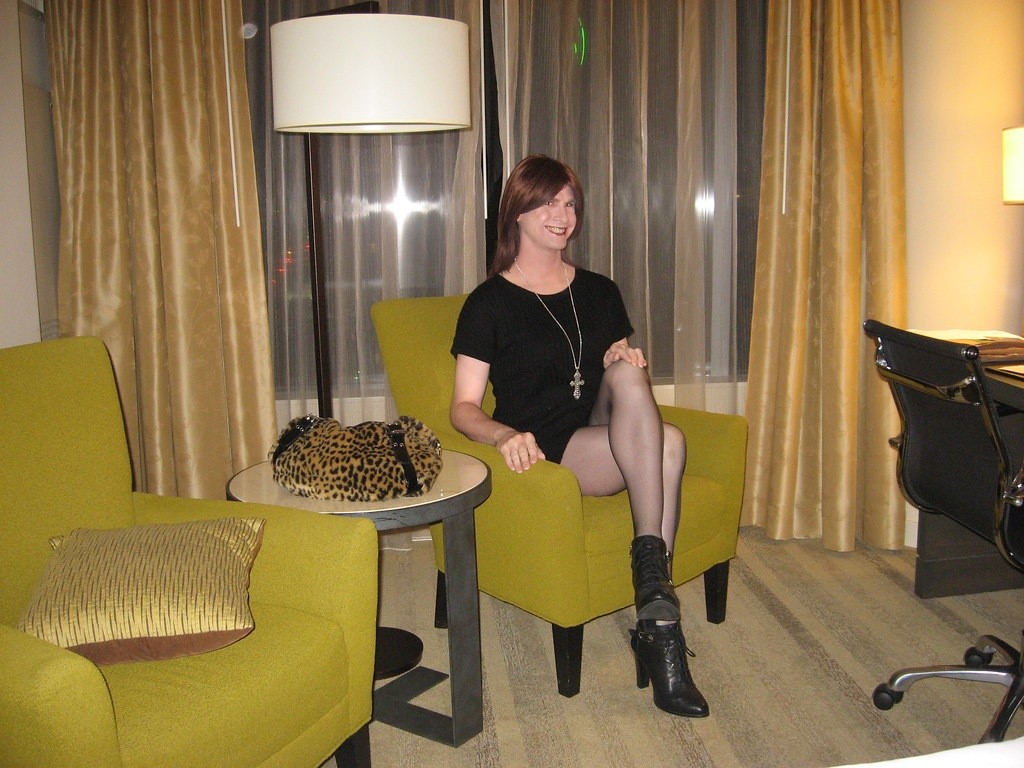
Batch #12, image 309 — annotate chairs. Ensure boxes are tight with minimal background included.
[855,317,1024,741]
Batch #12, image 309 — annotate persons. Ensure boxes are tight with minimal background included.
[448,153,710,722]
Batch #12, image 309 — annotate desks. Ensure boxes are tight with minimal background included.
[222,447,494,749]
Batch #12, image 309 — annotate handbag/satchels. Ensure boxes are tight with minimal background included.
[269,415,444,502]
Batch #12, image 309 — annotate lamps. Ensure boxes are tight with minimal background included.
[258,1,471,429]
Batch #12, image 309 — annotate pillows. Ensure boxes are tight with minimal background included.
[21,514,264,665]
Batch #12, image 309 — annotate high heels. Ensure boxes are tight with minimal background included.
[629,534,682,622]
[630,619,709,719]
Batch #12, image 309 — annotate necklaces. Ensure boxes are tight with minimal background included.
[511,255,585,400]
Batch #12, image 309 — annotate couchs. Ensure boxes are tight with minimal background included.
[0,334,377,768]
[369,291,751,689]
